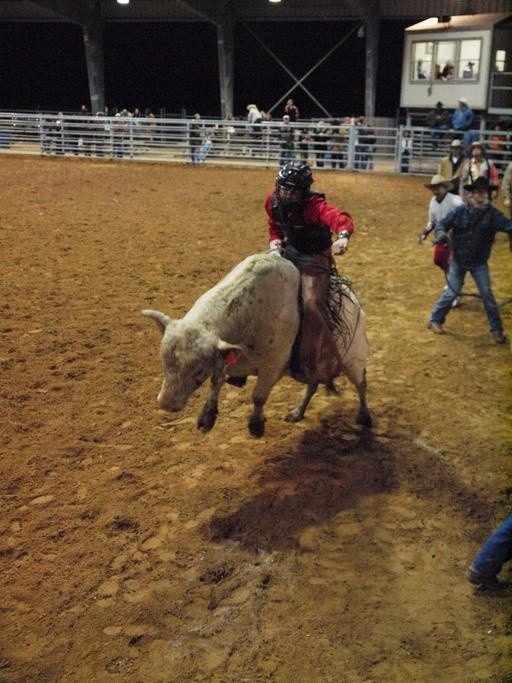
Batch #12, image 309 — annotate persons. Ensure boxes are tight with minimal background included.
[415,173,465,308]
[461,501,511,599]
[412,57,478,80]
[225,160,354,389]
[427,176,511,345]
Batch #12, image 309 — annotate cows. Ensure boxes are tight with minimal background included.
[141,254,371,437]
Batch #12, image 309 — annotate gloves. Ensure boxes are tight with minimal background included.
[418,226,431,245]
[433,232,448,250]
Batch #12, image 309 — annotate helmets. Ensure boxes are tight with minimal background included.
[275,160,316,194]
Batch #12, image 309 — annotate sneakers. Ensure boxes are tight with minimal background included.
[450,295,461,309]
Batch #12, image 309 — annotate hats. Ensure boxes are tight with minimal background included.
[245,103,256,110]
[447,139,465,151]
[462,176,498,193]
[467,140,486,155]
[283,114,291,119]
[422,174,456,192]
[456,98,469,109]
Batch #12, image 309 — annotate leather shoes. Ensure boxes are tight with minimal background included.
[428,321,444,335]
[492,330,506,344]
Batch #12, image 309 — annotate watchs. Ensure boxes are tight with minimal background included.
[337,231,350,239]
